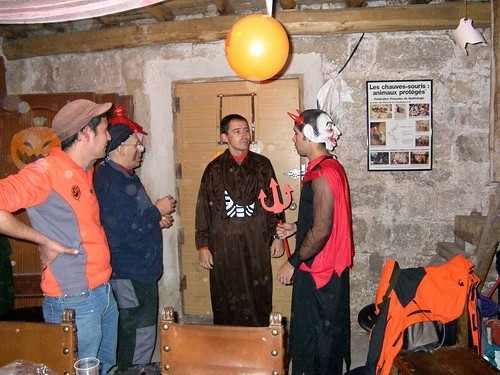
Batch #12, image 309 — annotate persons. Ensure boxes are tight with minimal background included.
[277,109,355,375]
[93,122,177,375]
[194,113,285,328]
[0,99,119,375]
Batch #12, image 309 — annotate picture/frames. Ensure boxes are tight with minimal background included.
[366,79,433,171]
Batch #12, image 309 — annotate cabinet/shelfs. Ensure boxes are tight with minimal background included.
[0,93,134,309]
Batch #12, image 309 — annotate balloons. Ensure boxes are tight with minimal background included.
[225,14,289,82]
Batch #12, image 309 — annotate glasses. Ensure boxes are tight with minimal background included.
[121,141,143,147]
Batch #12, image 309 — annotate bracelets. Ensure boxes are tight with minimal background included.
[273,234,280,240]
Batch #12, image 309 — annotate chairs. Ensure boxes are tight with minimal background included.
[384,254,500,375]
[159,307,286,375]
[0,309,80,375]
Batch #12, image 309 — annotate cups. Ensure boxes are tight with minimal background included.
[73,357,101,375]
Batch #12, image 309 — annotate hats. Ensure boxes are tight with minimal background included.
[107,123,134,156]
[51,98,113,142]
